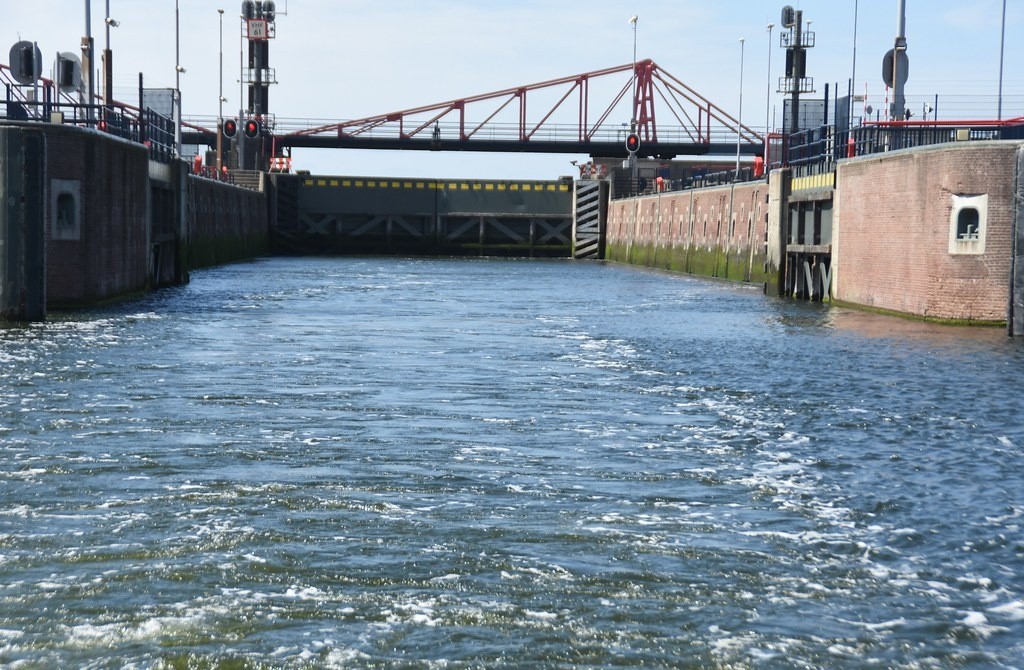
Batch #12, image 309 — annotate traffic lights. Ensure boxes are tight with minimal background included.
[626,133,641,154]
[224,119,238,138]
[245,119,259,139]
[906,109,913,120]
[925,103,933,114]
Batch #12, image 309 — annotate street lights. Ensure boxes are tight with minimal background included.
[804,18,813,45]
[629,13,640,197]
[733,39,750,184]
[103,0,118,124]
[216,7,225,180]
[174,0,185,91]
[760,23,775,176]
